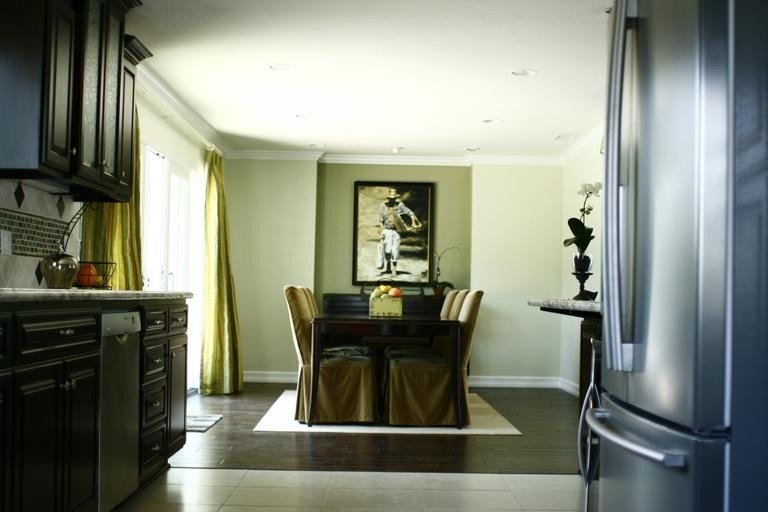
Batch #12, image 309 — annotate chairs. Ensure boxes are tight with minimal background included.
[283,285,484,428]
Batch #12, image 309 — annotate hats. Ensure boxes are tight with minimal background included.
[386,188,400,200]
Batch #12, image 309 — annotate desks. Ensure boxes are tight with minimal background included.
[526,298,603,480]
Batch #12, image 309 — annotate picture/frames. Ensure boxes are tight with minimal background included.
[351,180,435,287]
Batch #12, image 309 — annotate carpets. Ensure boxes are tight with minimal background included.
[253,389,524,436]
[186,413,224,431]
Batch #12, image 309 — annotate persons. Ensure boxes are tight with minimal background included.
[379,188,417,277]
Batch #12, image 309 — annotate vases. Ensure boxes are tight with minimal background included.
[570,252,594,271]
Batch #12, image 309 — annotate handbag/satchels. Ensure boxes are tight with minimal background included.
[376,239,386,269]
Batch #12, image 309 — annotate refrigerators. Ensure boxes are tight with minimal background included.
[576,0,767,512]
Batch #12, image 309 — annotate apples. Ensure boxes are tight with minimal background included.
[370,284,401,298]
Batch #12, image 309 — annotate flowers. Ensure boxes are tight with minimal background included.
[563,181,602,260]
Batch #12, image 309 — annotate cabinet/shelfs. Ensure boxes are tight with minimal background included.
[0,0,153,202]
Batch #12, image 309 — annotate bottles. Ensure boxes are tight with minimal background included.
[39,242,80,289]
